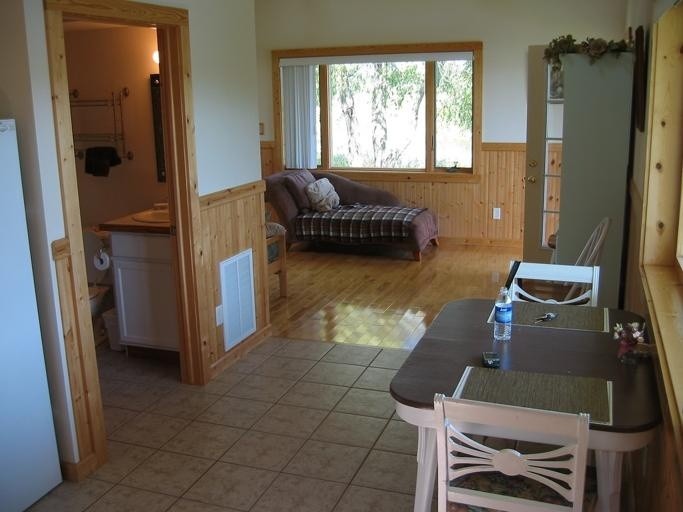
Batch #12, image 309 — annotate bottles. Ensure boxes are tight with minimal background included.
[494,285,512,340]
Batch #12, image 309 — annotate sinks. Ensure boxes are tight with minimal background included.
[132,210,171,223]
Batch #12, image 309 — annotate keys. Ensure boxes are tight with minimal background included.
[532,312,560,324]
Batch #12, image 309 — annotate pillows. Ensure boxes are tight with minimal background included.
[281,167,341,214]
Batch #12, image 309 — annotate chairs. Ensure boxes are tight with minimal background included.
[264,169,441,263]
[501,258,601,311]
[435,392,592,510]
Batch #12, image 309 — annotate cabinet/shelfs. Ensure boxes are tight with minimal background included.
[558,48,638,310]
[108,232,180,358]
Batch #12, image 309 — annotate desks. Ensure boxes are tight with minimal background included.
[390,297,664,512]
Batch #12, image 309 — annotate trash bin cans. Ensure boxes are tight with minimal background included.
[102,308,125,351]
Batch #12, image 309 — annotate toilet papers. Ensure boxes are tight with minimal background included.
[94,250,112,270]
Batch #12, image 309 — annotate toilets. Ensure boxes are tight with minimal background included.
[80,227,112,318]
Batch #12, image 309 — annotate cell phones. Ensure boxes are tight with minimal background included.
[482,352,501,368]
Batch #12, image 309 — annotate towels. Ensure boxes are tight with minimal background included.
[84,146,122,177]
